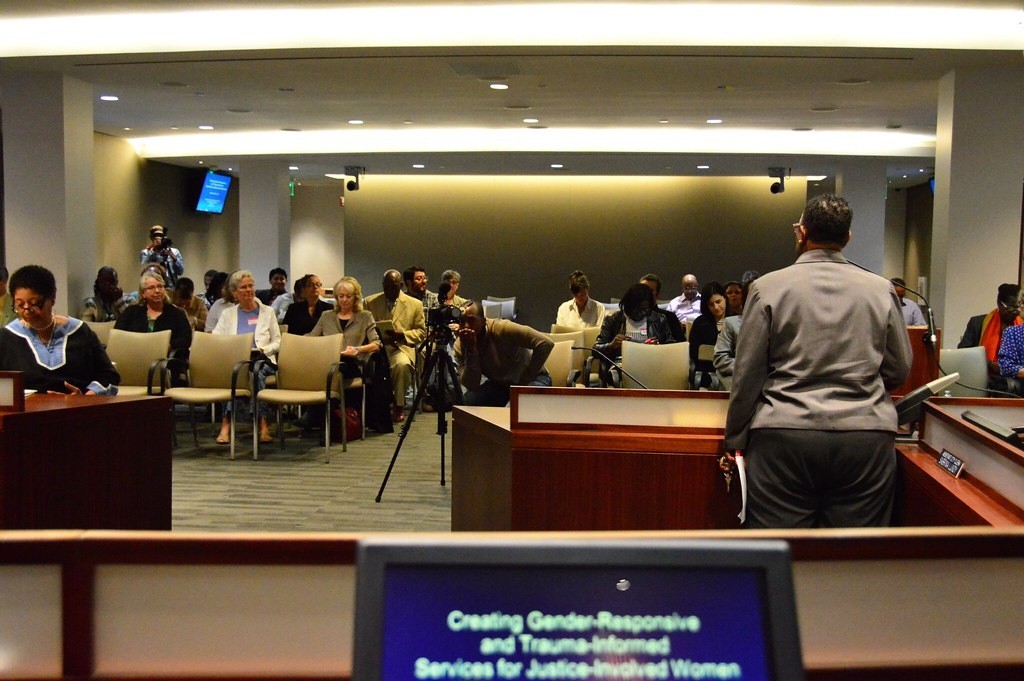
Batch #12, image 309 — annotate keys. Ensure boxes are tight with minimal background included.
[724,475,732,493]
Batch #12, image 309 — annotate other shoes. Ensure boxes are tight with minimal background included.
[261,434,271,443]
[216,435,229,443]
[293,410,320,428]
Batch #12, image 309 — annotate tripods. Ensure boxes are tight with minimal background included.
[375,322,466,502]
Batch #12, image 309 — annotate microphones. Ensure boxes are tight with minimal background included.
[571,346,648,389]
[439,282,452,295]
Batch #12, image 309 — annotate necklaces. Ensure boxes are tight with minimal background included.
[22,313,54,329]
[716,321,724,325]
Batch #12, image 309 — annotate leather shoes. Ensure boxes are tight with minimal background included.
[393,402,405,421]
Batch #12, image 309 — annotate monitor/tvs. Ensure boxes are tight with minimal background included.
[195,171,233,215]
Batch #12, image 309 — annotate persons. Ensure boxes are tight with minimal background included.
[271,275,334,339]
[719,191,912,529]
[255,268,291,307]
[432,269,470,403]
[957,282,1024,377]
[640,274,663,301]
[591,282,687,387]
[362,267,427,421]
[665,273,703,324]
[195,269,262,335]
[0,262,122,395]
[140,224,184,287]
[555,269,606,328]
[455,299,555,407]
[81,260,193,387]
[402,266,440,412]
[293,274,384,447]
[210,268,282,444]
[166,278,209,332]
[891,277,926,327]
[688,272,760,389]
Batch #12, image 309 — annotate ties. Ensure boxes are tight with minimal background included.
[388,302,394,312]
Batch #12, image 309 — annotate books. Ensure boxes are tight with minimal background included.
[374,319,395,344]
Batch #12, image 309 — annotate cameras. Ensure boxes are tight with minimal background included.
[161,227,172,247]
[428,305,461,322]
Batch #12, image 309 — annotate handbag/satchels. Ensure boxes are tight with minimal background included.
[330,400,361,441]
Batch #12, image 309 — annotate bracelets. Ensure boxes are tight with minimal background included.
[193,316,199,326]
[354,346,359,356]
[603,343,607,355]
[394,338,397,344]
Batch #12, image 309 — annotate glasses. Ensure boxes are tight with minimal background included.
[1001,301,1021,312]
[142,284,164,291]
[11,297,48,314]
[414,277,429,281]
[304,282,322,287]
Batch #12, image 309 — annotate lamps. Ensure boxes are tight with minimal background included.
[768,167,792,194]
[343,165,366,191]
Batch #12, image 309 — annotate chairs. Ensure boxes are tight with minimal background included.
[551,324,608,387]
[342,350,384,441]
[85,321,117,349]
[937,346,989,397]
[250,332,348,466]
[527,339,582,387]
[540,332,594,387]
[161,330,255,462]
[697,344,716,390]
[611,340,702,390]
[481,296,518,320]
[105,328,177,447]
